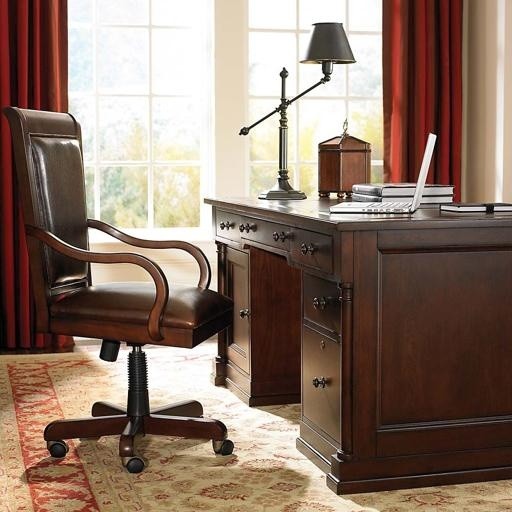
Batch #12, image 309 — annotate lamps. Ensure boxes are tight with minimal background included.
[237,20,357,200]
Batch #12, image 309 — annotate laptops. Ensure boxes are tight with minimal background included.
[329,132,437,214]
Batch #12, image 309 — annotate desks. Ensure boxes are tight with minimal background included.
[200,195,512,495]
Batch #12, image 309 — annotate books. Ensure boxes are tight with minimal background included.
[329,201,422,214]
[351,193,454,204]
[352,183,455,196]
[439,202,512,213]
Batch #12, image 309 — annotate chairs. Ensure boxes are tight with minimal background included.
[0,103,237,472]
